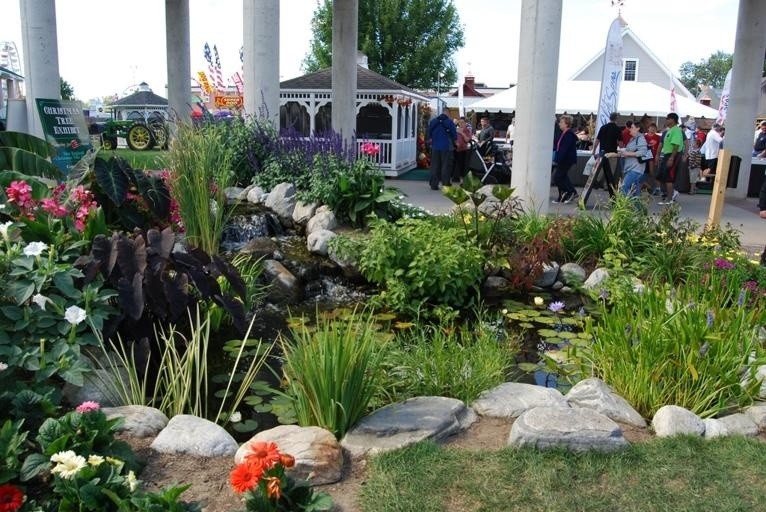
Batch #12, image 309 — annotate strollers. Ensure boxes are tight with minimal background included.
[463,140,512,184]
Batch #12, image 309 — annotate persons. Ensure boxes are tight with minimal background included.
[747,121,766,218]
[551,111,726,206]
[95,107,104,136]
[425,107,515,191]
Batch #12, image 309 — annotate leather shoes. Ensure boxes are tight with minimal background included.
[430,183,452,190]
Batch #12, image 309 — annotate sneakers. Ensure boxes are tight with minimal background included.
[551,189,579,205]
[656,189,680,206]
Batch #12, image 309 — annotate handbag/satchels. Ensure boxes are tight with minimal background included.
[638,148,655,164]
